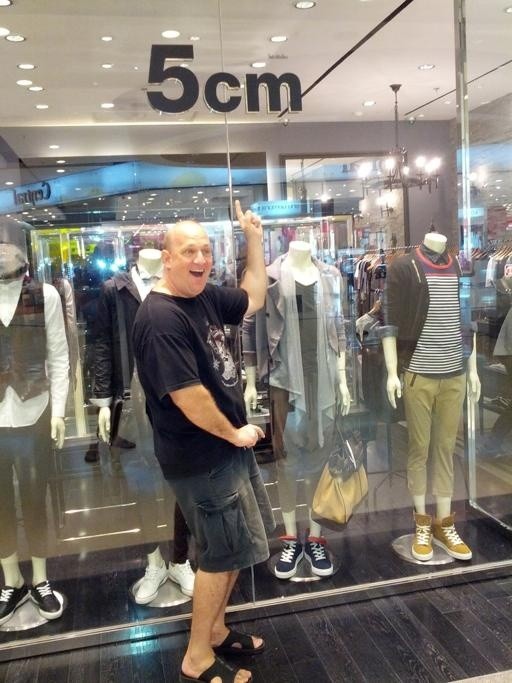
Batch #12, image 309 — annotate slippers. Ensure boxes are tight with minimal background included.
[179,655,254,683]
[212,629,267,655]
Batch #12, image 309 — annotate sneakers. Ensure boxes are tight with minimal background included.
[108,435,136,449]
[135,561,169,604]
[274,536,303,579]
[169,560,196,597]
[85,445,100,462]
[304,528,333,576]
[30,580,63,619]
[0,581,31,626]
[432,512,472,561]
[411,506,434,561]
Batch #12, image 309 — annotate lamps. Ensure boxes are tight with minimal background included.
[356,174,400,219]
[353,78,446,196]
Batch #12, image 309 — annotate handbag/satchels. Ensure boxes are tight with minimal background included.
[99,401,160,478]
[311,431,369,531]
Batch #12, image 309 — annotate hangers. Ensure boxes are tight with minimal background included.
[350,231,512,315]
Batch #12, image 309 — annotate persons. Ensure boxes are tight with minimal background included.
[92,247,195,604]
[0,242,70,627]
[376,231,482,562]
[242,240,352,578]
[128,198,270,682]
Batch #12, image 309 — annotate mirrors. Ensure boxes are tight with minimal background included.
[277,148,412,257]
